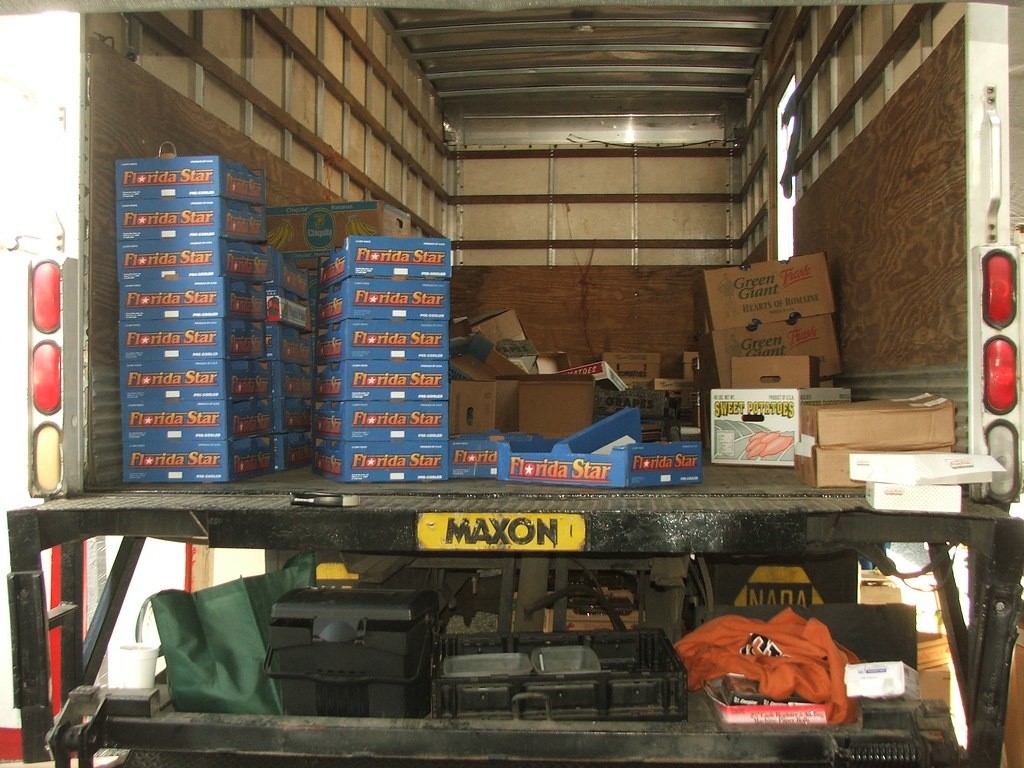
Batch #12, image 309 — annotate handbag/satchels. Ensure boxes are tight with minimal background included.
[134,552,317,717]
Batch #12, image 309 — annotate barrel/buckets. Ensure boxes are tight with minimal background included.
[106,642,160,688]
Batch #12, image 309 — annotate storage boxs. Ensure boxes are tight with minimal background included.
[262,588,438,718]
[114,156,956,489]
[431,629,688,723]
[844,661,921,701]
[702,678,828,733]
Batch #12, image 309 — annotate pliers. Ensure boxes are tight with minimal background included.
[291,490,361,506]
